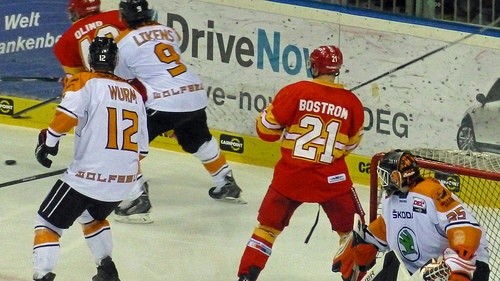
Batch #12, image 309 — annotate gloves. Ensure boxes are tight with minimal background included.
[35,129,59,168]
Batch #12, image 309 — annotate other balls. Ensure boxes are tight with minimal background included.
[3,158,18,166]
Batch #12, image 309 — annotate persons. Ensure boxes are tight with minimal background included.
[112,0,247,223]
[33,36,150,281]
[54,0,149,104]
[237,45,367,281]
[332,149,490,281]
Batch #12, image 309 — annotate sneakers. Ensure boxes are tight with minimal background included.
[91,264,121,281]
[32,271,56,281]
[114,195,154,224]
[208,176,248,204]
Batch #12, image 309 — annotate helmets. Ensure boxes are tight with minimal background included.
[119,0,148,20]
[89,37,119,70]
[310,46,343,76]
[67,0,100,15]
[378,149,420,193]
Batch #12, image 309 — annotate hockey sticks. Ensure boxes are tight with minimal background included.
[350,17,500,92]
[0,166,68,188]
[9,94,63,119]
